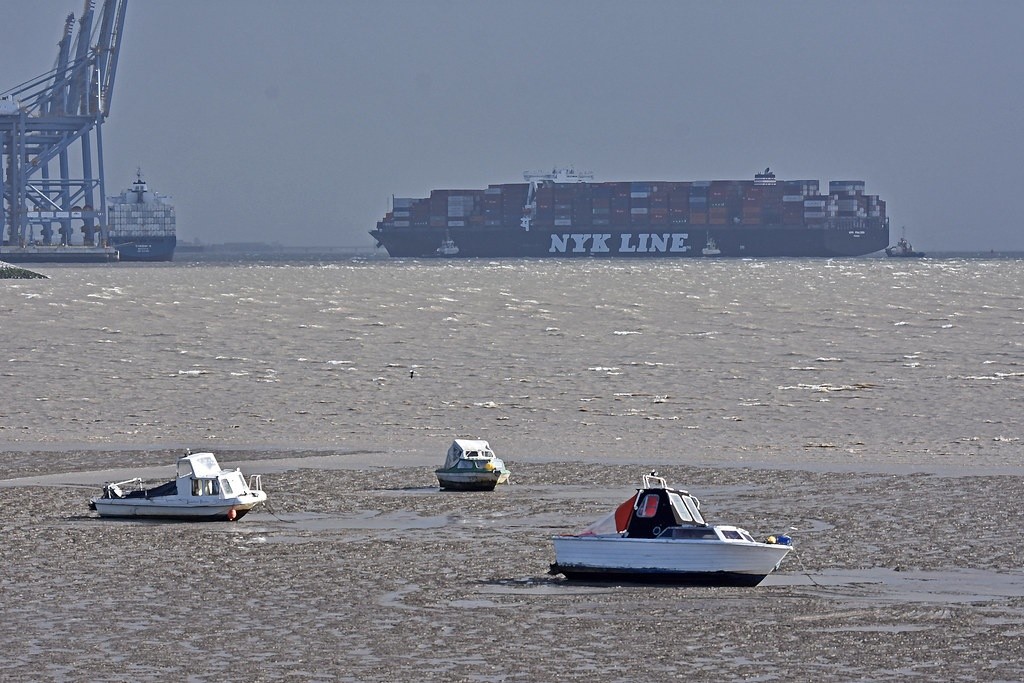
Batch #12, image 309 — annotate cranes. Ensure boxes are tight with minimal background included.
[0,0,130,246]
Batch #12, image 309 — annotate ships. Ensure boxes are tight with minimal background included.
[106,167,178,265]
[366,168,892,260]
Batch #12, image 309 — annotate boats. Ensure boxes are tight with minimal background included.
[551,469,795,588]
[432,440,511,492]
[87,448,267,522]
[885,238,925,260]
